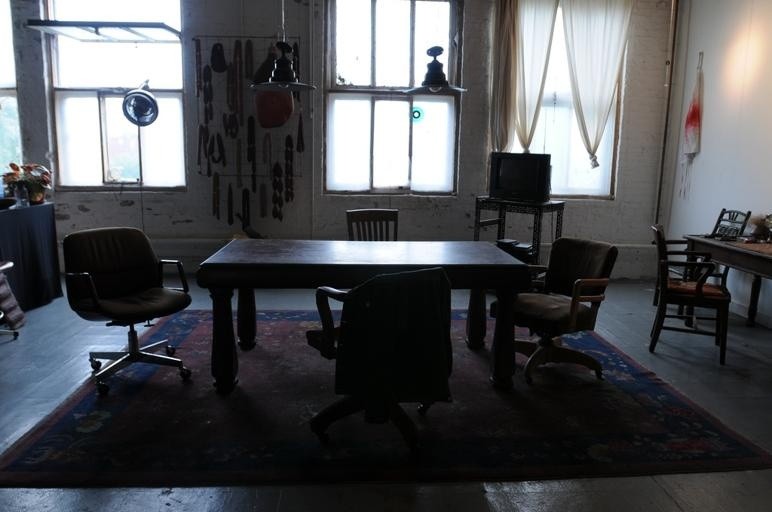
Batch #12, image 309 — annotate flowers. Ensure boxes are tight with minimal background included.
[1,161,53,194]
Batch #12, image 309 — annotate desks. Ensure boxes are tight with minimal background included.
[472,195,565,296]
[682,234,771,328]
[196,238,529,397]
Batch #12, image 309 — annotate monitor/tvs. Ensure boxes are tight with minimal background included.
[490,151,551,202]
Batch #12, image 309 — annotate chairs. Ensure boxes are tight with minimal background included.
[307,266,454,447]
[491,237,618,385]
[63,227,193,394]
[345,208,399,241]
[649,208,750,366]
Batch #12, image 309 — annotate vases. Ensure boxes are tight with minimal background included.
[27,185,46,204]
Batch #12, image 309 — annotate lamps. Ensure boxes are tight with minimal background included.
[250,1,318,95]
[404,46,467,96]
[122,80,158,231]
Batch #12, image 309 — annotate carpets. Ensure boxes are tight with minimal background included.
[0,311,771,488]
